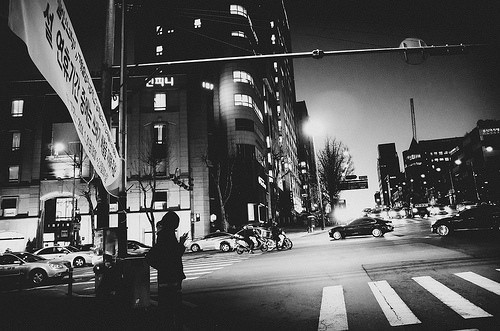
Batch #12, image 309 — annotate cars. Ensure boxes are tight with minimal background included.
[429,205,496,238]
[31,245,96,267]
[187,232,232,252]
[325,216,394,240]
[127,238,152,256]
[1,251,69,286]
[355,203,494,222]
[214,226,269,253]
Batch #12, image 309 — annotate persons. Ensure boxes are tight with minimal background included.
[154,212,187,331]
[211,212,319,254]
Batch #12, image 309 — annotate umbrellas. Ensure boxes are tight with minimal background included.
[307,216,316,218]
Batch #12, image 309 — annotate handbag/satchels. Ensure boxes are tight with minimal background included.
[146,243,161,269]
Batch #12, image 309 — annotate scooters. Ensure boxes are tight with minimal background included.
[234,232,269,255]
[262,233,293,250]
